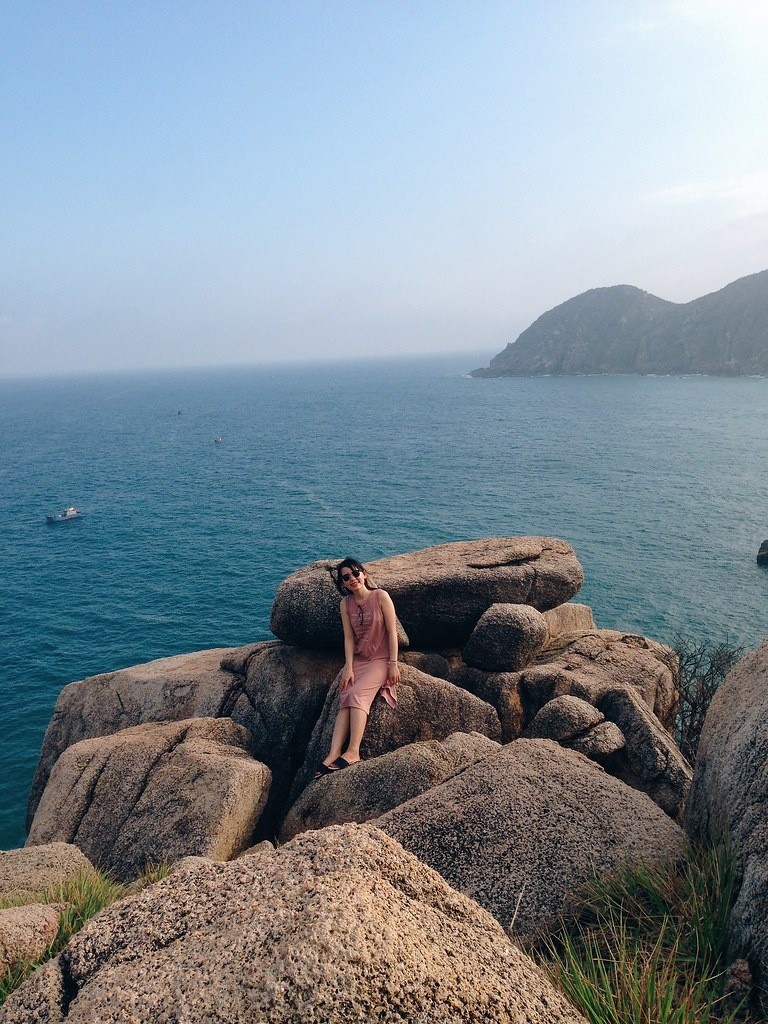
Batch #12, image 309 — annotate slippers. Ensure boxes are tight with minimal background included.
[328,756,351,771]
[314,762,329,780]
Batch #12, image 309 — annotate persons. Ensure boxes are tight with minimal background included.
[315,558,401,777]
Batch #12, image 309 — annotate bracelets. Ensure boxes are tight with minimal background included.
[390,660,397,662]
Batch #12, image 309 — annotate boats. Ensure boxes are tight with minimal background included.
[44,506,83,522]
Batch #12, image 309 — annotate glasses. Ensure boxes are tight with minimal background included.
[341,569,361,582]
